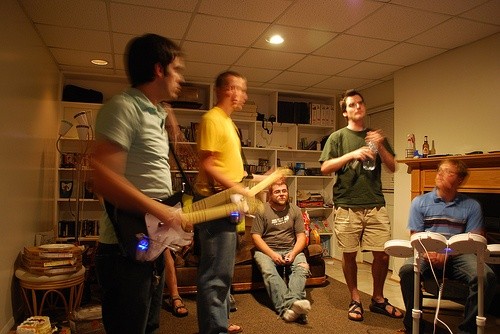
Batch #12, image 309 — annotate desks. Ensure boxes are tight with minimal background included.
[15,264,86,334]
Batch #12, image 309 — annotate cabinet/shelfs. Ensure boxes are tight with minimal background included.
[54,71,337,257]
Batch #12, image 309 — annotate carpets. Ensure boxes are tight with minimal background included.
[7,274,500,334]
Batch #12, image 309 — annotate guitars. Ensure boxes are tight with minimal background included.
[229,168,292,214]
[117,178,267,262]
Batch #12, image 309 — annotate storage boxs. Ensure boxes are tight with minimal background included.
[278,101,334,125]
[230,104,257,121]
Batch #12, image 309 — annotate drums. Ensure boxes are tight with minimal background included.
[382,239,415,258]
[447,234,488,255]
[485,244,500,265]
[14,261,85,327]
[411,231,448,252]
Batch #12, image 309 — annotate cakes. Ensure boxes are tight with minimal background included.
[17,316,52,334]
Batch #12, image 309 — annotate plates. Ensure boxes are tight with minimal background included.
[165,101,203,110]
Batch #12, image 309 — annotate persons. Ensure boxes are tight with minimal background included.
[162,246,187,318]
[194,72,285,334]
[400,158,496,334]
[251,181,312,321]
[89,34,191,334]
[319,89,404,322]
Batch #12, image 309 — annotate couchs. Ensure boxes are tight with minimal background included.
[164,217,327,295]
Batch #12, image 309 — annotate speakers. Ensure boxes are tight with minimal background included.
[278,101,309,124]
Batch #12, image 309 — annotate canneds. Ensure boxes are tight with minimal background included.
[301,137,307,149]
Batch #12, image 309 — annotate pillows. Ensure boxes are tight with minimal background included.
[302,210,310,246]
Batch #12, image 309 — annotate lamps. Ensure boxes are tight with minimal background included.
[57,111,94,246]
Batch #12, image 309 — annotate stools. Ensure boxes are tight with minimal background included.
[422,290,465,318]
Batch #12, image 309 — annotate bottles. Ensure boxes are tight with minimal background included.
[362,141,378,171]
[422,136,429,158]
[405,139,414,159]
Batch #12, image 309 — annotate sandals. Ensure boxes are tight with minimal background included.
[227,323,242,333]
[348,299,364,321]
[369,297,403,318]
[169,296,187,316]
